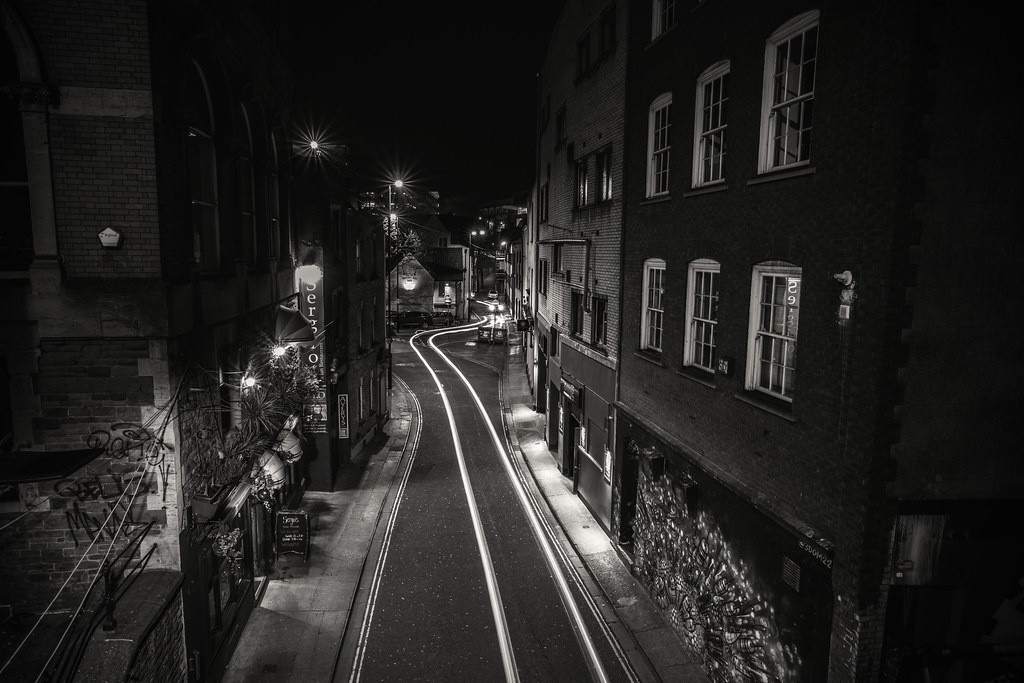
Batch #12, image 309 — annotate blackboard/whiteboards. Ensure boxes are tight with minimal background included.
[274,511,310,555]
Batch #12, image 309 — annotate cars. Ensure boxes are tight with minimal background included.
[488,289,504,313]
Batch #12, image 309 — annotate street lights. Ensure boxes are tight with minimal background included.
[387,212,402,334]
[386,178,408,390]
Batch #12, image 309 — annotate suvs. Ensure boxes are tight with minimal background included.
[388,311,433,329]
[427,311,454,326]
